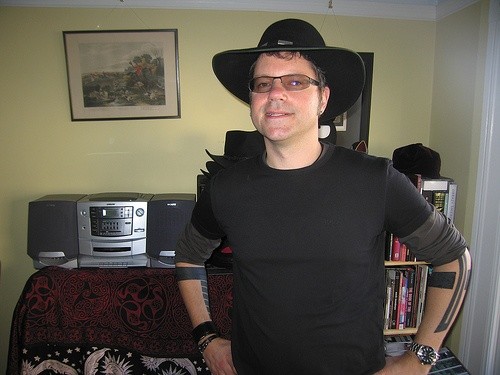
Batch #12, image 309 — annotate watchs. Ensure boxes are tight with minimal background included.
[408,342,440,365]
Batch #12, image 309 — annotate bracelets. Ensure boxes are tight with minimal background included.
[191,321,218,345]
[199,335,221,353]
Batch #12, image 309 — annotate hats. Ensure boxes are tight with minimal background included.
[211,18,366,122]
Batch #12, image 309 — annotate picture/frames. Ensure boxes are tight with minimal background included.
[332,51,374,151]
[62,28,181,122]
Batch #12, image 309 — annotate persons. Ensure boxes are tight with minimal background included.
[174,18,471,375]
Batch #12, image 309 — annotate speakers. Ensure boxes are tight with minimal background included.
[26,194,87,269]
[146,193,197,268]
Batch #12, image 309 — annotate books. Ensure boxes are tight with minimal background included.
[384,264,434,330]
[384,335,414,357]
[384,174,457,262]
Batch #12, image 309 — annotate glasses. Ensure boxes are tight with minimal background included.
[247,74,319,93]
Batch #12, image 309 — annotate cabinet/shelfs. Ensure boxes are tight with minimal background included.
[383,261,434,337]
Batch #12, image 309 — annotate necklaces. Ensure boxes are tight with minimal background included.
[316,140,324,161]
